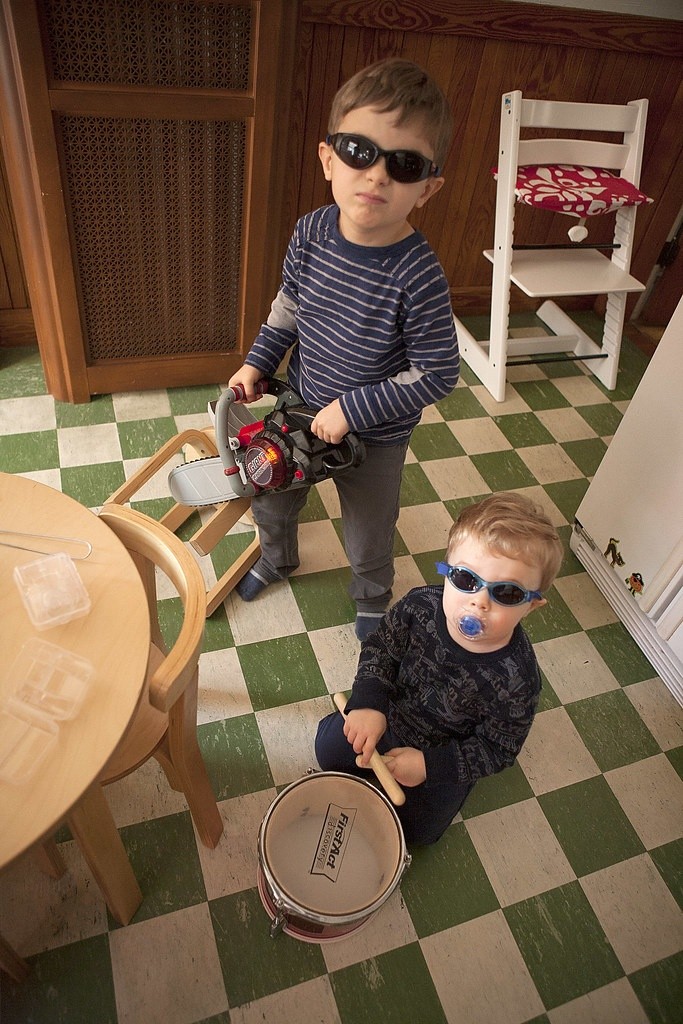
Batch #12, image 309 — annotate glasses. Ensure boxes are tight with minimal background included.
[436,561,543,607]
[325,132,439,185]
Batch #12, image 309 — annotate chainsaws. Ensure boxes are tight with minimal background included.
[167,386,368,511]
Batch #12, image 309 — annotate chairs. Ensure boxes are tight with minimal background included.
[31,504,223,926]
[450,90,649,404]
[0,472,151,981]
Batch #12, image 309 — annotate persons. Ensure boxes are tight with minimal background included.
[228,57,460,643]
[316,492,566,851]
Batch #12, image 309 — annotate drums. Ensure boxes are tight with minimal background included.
[247,763,418,955]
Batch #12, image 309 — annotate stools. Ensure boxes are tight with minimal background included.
[103,426,262,618]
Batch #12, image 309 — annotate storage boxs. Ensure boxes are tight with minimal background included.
[14,550,91,630]
[0,637,96,785]
